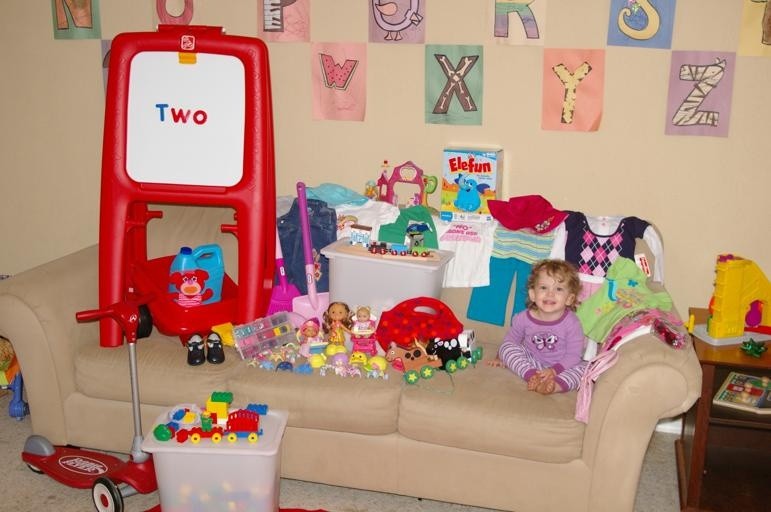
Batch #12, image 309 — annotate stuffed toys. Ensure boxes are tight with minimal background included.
[168,269,213,307]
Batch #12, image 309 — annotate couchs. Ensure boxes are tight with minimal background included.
[0,202,701,511]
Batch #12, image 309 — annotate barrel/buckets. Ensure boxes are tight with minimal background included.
[169,244,224,306]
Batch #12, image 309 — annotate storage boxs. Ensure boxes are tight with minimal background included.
[317,236,454,325]
[138,406,289,512]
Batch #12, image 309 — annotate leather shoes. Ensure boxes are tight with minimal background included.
[187,335,205,366]
[206,332,225,364]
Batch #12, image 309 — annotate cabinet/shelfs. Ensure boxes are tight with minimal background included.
[674,309,771,510]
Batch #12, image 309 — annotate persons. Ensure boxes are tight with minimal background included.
[298,302,374,358]
[489,256,594,396]
